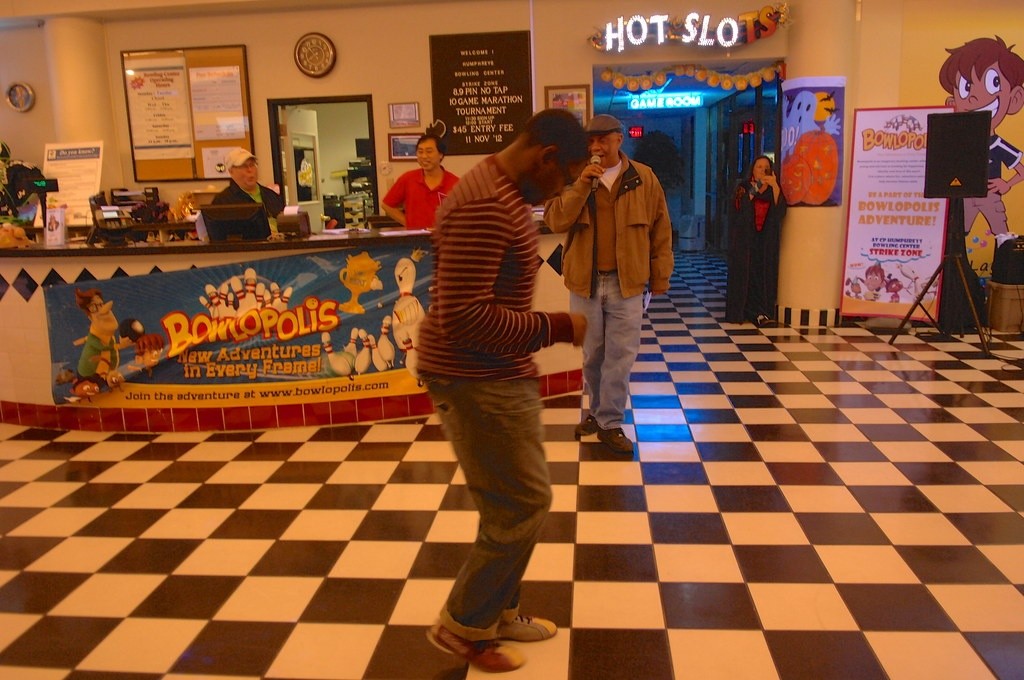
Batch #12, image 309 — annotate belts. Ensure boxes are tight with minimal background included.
[595,269,620,277]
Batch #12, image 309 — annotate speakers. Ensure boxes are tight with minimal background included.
[924,110,993,198]
[991,235,1024,285]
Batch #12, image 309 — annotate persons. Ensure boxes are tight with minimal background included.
[211,148,286,232]
[542,114,673,453]
[381,134,459,230]
[733,155,786,327]
[413,107,594,675]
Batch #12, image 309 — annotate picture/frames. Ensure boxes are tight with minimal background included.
[545,84,591,130]
[388,133,426,162]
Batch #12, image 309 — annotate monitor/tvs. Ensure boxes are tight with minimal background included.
[200,203,272,241]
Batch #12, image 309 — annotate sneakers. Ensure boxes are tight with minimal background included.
[426,619,528,672]
[498,614,558,642]
[574,415,596,435]
[597,425,634,453]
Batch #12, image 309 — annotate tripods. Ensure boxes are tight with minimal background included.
[888,196,991,356]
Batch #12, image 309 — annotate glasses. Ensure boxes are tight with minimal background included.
[237,162,259,172]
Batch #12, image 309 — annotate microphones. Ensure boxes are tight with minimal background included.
[590,155,601,192]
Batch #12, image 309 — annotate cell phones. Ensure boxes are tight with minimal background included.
[765,168,773,176]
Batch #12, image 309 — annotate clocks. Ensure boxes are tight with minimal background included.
[293,32,337,78]
[5,81,36,114]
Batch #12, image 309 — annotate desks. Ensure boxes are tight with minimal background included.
[17,221,194,244]
[346,167,372,195]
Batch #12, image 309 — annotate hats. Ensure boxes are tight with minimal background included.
[583,114,625,135]
[225,148,257,168]
